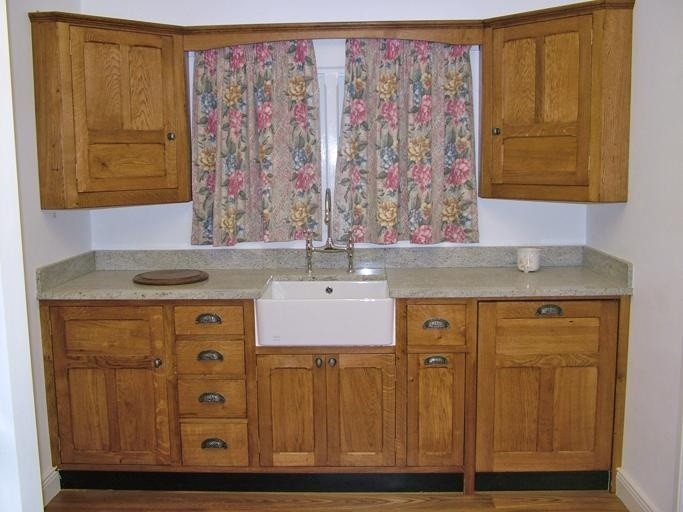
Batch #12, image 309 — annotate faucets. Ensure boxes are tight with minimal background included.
[323,187,332,240]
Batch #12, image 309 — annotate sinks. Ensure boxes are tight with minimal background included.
[255,281,396,346]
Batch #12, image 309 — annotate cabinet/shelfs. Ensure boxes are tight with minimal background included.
[31,21,192,210]
[38,297,632,495]
[478,9,632,202]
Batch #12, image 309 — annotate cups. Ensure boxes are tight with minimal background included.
[517,248,540,272]
